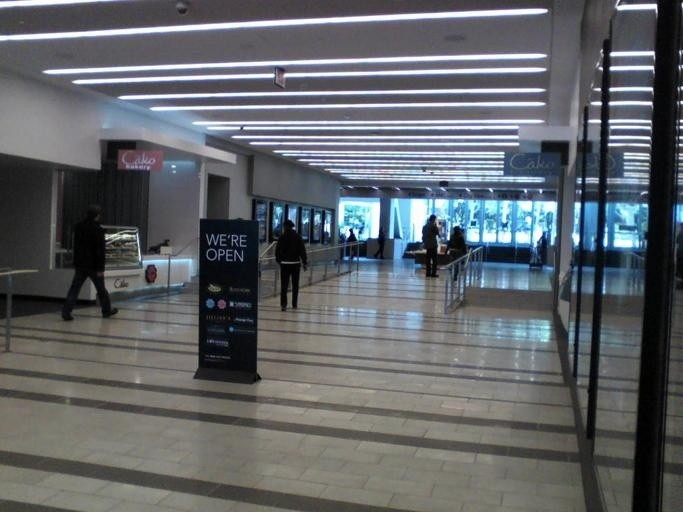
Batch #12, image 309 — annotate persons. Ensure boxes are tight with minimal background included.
[58,204,119,320]
[273,219,307,311]
[421,215,440,280]
[536,230,546,265]
[346,228,358,260]
[444,224,464,282]
[373,226,386,260]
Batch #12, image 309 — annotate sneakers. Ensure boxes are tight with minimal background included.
[61,310,73,321]
[430,274,439,278]
[101,308,118,318]
[281,304,287,312]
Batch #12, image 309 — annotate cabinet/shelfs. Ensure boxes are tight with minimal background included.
[91,224,141,304]
